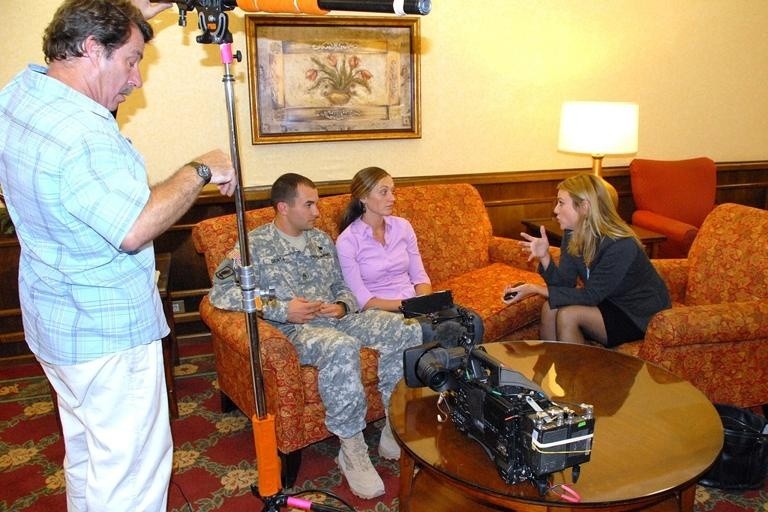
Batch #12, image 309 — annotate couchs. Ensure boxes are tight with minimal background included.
[192,183,583,453]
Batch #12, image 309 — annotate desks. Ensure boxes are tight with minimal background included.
[49,253,179,419]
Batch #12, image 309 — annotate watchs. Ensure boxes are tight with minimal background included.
[184,159,212,187]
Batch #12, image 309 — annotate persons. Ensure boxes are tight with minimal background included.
[0,1,237,512]
[336,167,484,346]
[500,173,672,349]
[207,172,425,499]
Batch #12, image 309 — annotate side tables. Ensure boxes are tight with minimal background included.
[522,219,666,258]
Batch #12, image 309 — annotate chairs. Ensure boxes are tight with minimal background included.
[630,158,716,257]
[617,203,768,410]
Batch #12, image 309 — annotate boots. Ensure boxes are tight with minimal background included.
[335,433,385,499]
[378,409,401,460]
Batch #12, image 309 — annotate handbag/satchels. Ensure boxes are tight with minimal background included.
[697,404,768,490]
[401,289,454,318]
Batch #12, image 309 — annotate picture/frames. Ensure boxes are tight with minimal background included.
[244,12,424,145]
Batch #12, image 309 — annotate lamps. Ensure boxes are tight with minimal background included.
[557,101,639,175]
